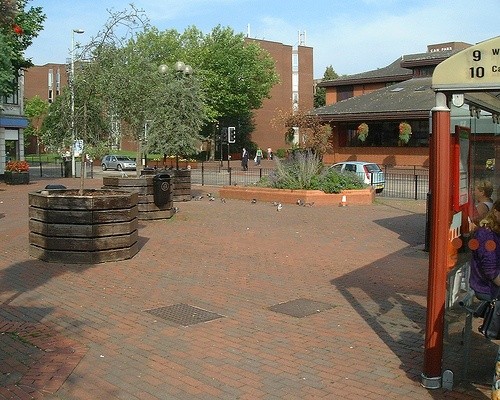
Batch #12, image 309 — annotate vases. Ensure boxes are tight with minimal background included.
[3,171,30,185]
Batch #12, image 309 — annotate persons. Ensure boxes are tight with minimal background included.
[254,148,263,166]
[241,147,249,172]
[469,201,500,340]
[474,181,495,218]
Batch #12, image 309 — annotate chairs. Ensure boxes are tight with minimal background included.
[345,164,353,172]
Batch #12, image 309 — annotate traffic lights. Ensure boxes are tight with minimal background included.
[227,127,236,143]
[220,127,228,142]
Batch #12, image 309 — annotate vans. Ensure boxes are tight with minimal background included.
[328,161,385,193]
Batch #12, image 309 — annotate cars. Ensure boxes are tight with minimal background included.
[100,154,137,171]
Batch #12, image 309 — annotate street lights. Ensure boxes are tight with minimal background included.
[157,62,193,172]
[71,29,84,178]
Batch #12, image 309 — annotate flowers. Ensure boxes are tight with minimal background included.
[399,121,413,145]
[6,160,30,173]
[357,122,370,142]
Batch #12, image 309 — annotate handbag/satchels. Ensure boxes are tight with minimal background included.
[474,298,500,341]
